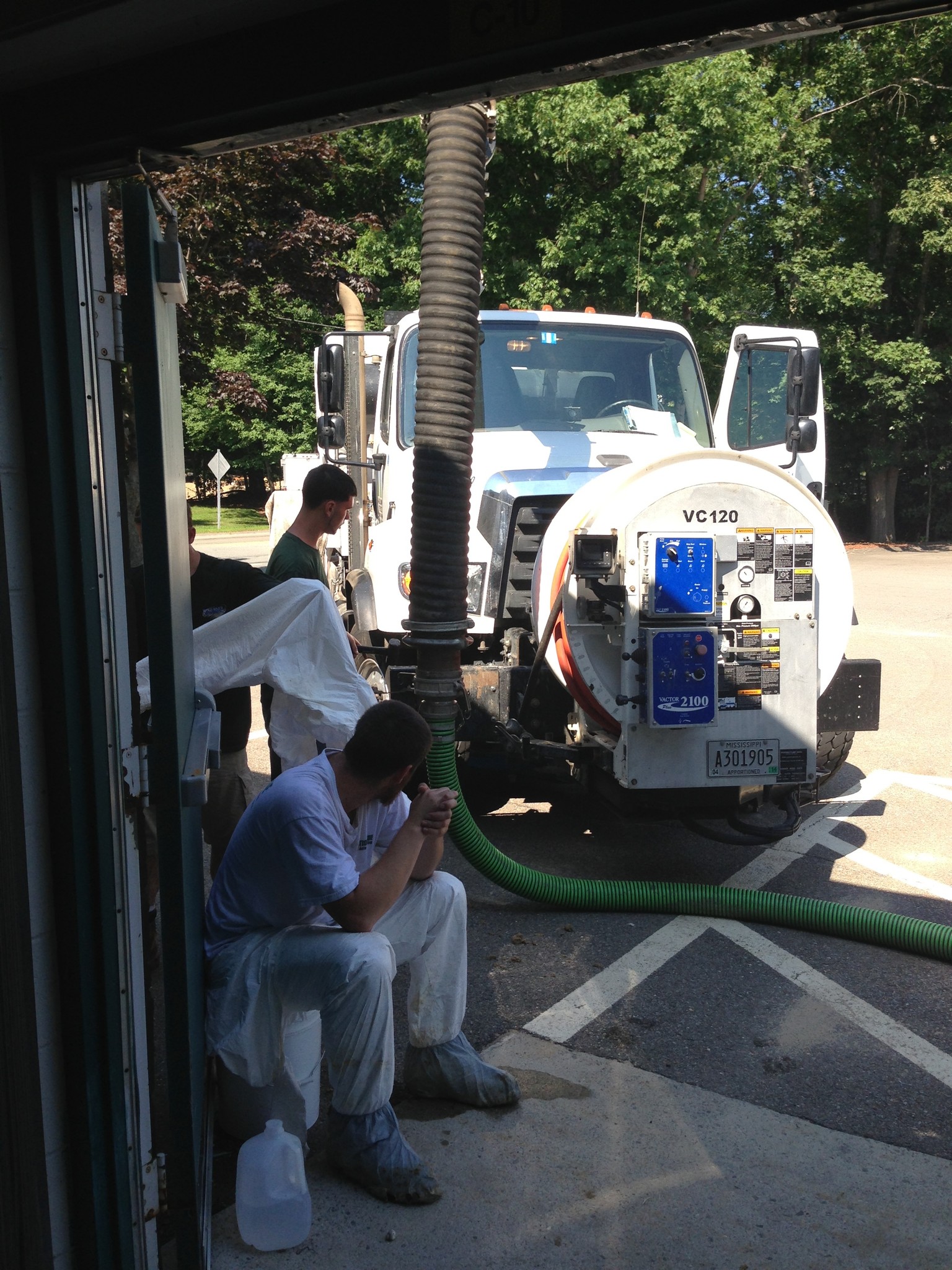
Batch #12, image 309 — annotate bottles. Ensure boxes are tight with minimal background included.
[234,1117,311,1252]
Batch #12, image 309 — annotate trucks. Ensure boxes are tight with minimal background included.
[255,282,886,844]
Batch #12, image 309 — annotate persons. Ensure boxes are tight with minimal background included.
[260,463,368,781]
[129,496,332,975]
[203,698,520,1207]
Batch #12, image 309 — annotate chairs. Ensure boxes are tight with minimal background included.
[573,376,615,419]
